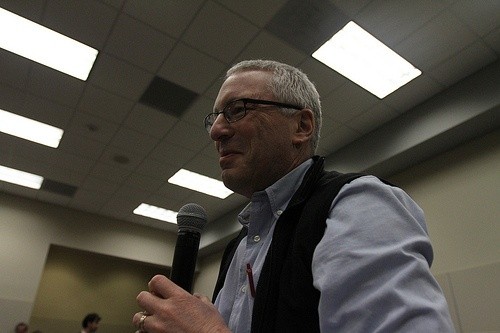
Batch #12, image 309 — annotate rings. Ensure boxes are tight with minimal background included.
[139,310,152,333]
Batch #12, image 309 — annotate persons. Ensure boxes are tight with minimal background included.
[131,59,456,333]
[77,312,102,333]
[13,322,29,333]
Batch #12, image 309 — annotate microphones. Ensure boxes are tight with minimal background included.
[171,203,208,295]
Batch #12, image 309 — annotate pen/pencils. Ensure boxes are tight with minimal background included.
[245,263,257,298]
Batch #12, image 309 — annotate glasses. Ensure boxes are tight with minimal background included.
[204,98,304,131]
